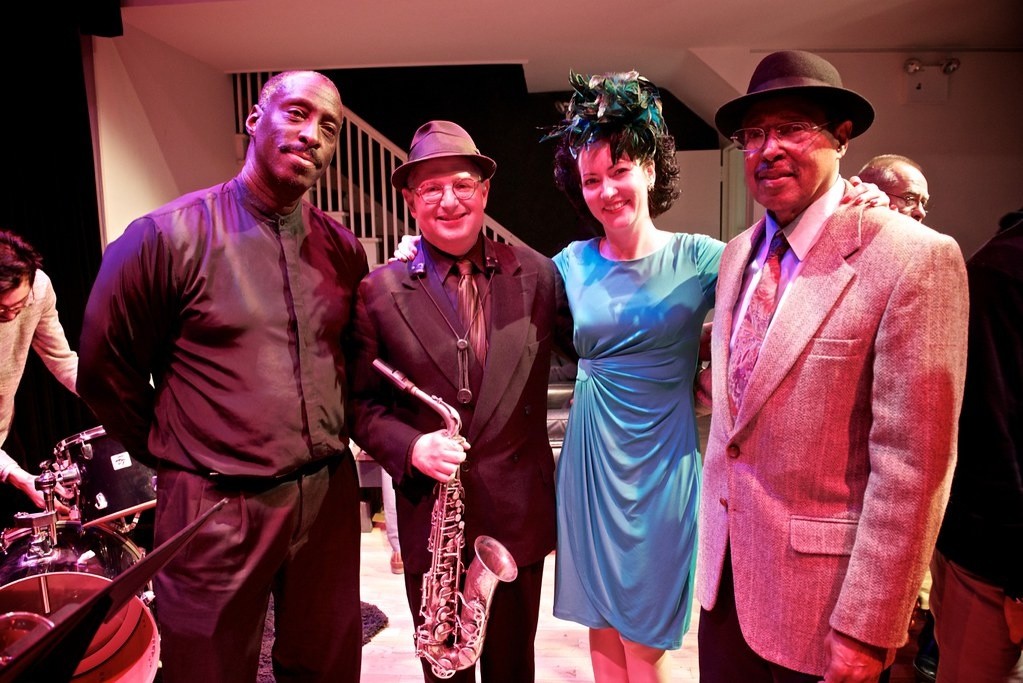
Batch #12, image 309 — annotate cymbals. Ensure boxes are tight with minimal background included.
[0,495,233,683]
[80,499,158,529]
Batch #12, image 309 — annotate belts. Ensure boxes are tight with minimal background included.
[203,452,345,495]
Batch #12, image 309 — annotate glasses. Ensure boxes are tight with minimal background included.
[405,177,485,205]
[0,287,36,313]
[886,193,929,209]
[729,121,835,151]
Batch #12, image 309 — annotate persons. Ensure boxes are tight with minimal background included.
[0,230,79,515]
[854,155,1023,683]
[692,49,969,683]
[345,120,714,683]
[76,69,371,683]
[388,71,889,683]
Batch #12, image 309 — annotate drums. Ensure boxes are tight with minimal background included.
[55,423,158,528]
[0,519,164,682]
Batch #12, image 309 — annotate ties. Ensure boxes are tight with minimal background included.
[726,236,790,428]
[454,258,486,372]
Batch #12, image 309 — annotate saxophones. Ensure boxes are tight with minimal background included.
[369,357,518,681]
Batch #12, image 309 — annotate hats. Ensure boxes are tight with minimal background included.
[391,120,498,190]
[714,50,875,141]
